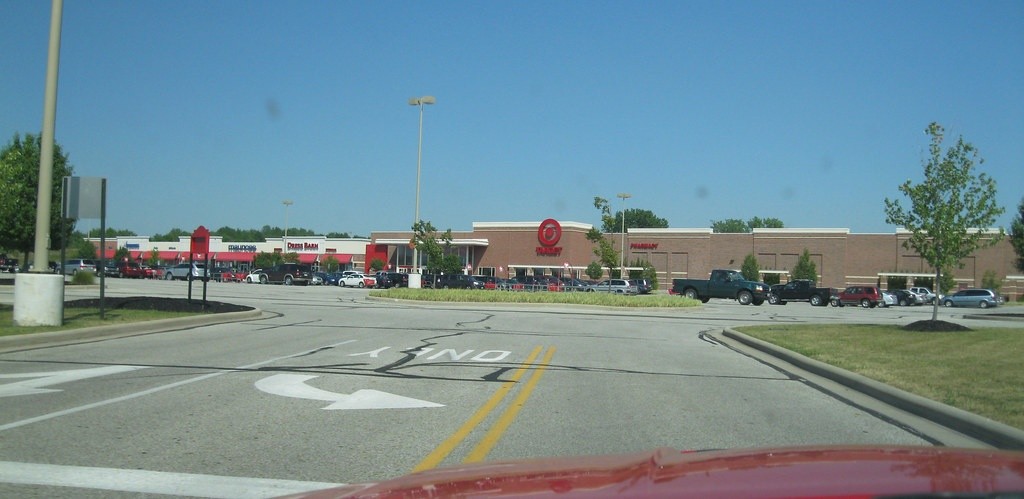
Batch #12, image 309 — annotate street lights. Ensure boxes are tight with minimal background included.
[408,97,436,288]
[282,200,293,260]
[617,193,631,280]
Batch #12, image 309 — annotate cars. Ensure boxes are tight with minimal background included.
[210,267,246,283]
[635,278,652,294]
[315,270,441,288]
[910,287,945,306]
[143,265,164,279]
[436,274,484,289]
[308,273,323,285]
[484,276,525,292]
[902,290,925,306]
[246,269,263,283]
[49,261,58,269]
[877,291,897,308]
[96,264,118,278]
[509,274,598,293]
[884,289,916,306]
[338,274,377,288]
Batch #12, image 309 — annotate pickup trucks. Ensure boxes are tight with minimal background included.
[0,253,19,273]
[768,279,846,306]
[668,270,772,306]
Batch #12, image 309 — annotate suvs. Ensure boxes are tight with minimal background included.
[583,279,638,296]
[117,261,146,279]
[838,285,885,308]
[259,264,313,286]
[165,263,210,282]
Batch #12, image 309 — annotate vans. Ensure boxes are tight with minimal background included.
[939,289,997,309]
[54,258,97,277]
